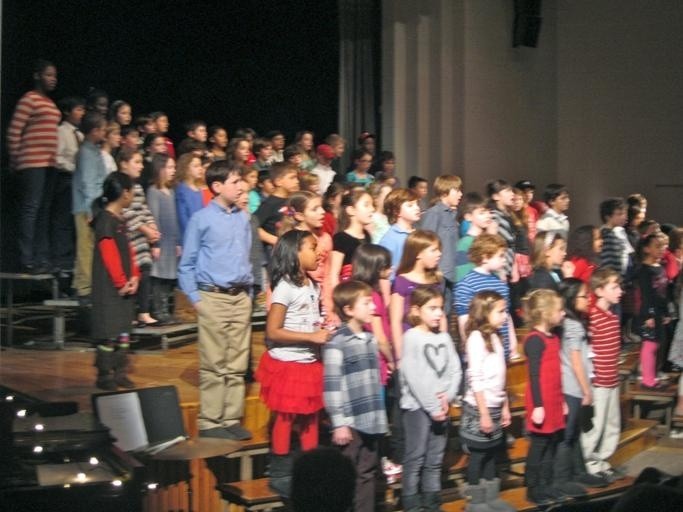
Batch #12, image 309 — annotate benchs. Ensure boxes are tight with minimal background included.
[1,266,683,512]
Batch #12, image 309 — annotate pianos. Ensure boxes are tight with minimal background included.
[0,382,146,512]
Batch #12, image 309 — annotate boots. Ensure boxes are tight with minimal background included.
[552,460,588,496]
[423,492,443,512]
[570,442,608,488]
[402,495,424,512]
[268,453,296,495]
[486,478,516,512]
[537,462,565,502]
[112,345,135,388]
[132,285,196,327]
[524,464,548,503]
[464,480,496,512]
[94,346,118,391]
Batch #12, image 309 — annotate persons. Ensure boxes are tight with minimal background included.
[5,58,683,512]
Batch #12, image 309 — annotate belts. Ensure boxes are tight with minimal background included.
[198,286,248,295]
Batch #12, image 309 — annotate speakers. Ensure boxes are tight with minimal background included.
[514,0,541,48]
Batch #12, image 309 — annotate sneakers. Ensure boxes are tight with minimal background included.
[22,257,94,310]
[621,331,683,439]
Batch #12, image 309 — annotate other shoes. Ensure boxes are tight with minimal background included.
[198,426,239,441]
[605,467,625,480]
[591,471,617,484]
[225,423,253,440]
[381,457,404,484]
